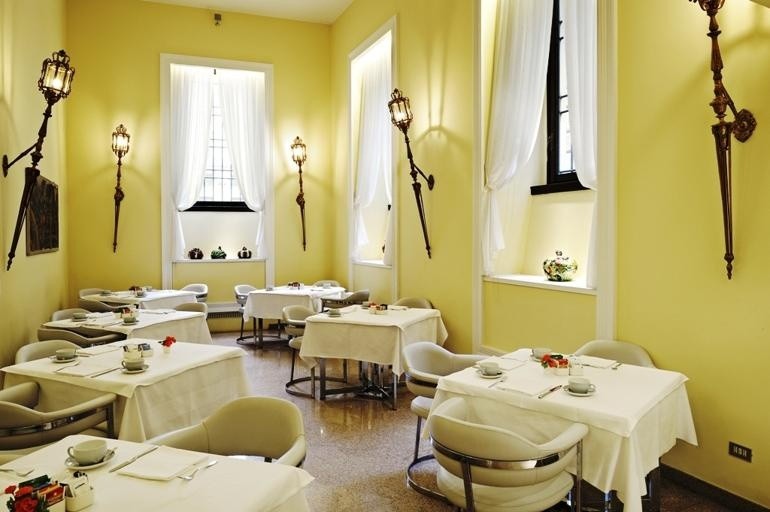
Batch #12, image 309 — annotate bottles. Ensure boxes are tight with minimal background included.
[543,251,578,281]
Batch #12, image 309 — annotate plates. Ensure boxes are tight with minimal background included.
[328,314,340,317]
[72,318,86,322]
[477,370,502,378]
[103,293,111,296]
[65,448,115,470]
[564,384,597,396]
[52,357,75,363]
[531,355,542,361]
[121,365,150,373]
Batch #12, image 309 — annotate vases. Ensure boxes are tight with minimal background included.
[541,248,579,283]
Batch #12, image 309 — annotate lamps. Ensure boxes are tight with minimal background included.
[290,134,312,254]
[687,0,757,281]
[380,84,436,260]
[2,45,78,274]
[106,121,130,253]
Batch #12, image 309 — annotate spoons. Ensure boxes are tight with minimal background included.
[56,360,80,371]
[612,363,621,369]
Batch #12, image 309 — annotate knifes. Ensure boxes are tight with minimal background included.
[92,367,120,378]
[488,377,506,388]
[109,446,158,473]
[538,384,561,399]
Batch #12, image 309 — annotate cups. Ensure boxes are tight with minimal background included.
[129,286,152,298]
[329,309,338,315]
[67,439,108,465]
[569,377,596,394]
[122,343,153,370]
[362,301,385,315]
[104,290,110,294]
[323,283,331,289]
[55,349,74,360]
[46,483,95,511]
[288,282,302,290]
[112,305,140,325]
[534,348,552,359]
[73,313,85,319]
[480,361,499,375]
